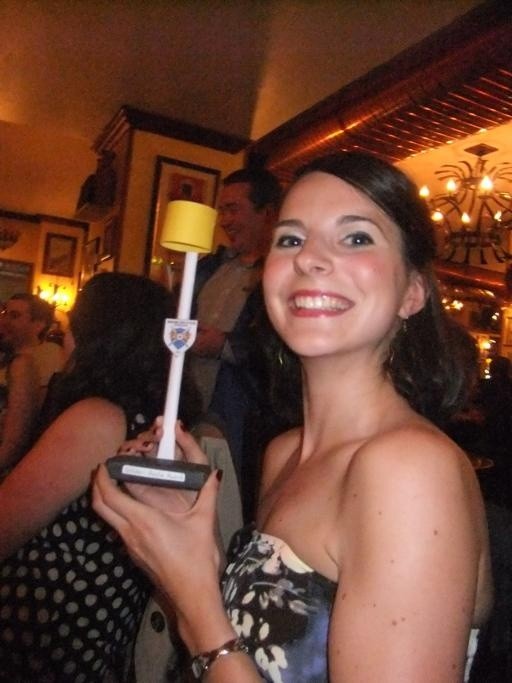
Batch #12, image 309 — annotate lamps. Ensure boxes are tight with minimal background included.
[418,142,512,266]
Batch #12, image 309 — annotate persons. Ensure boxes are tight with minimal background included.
[0,145,511,682]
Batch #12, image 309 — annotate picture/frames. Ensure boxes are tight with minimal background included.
[143,155,222,291]
[42,231,77,277]
[82,237,99,287]
[101,216,115,261]
